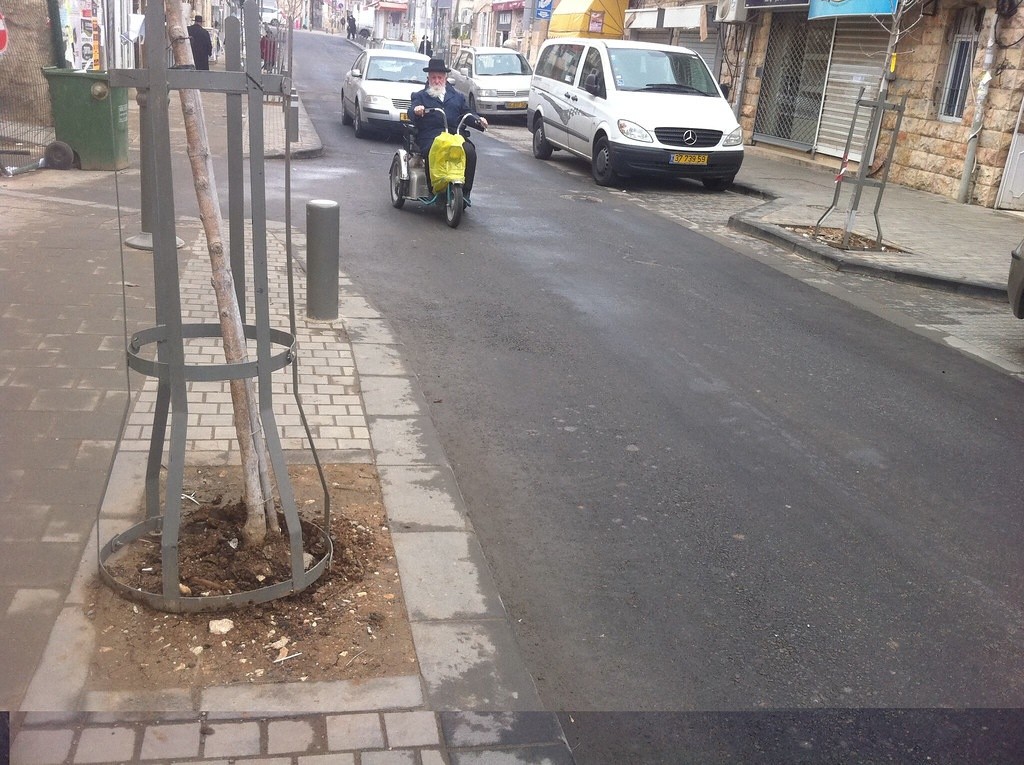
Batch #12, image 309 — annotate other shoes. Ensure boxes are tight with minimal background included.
[428,194,436,203]
[464,193,471,204]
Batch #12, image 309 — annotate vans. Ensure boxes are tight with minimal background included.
[526,38,744,193]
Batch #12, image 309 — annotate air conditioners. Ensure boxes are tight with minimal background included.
[714,0,747,22]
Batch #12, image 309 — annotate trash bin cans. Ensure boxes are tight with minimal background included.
[39,66,129,172]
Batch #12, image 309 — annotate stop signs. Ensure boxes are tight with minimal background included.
[0,15,9,55]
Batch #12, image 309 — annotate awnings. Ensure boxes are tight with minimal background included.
[548,0,629,39]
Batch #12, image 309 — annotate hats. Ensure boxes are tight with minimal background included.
[192,16,205,22]
[422,35,428,39]
[423,59,451,72]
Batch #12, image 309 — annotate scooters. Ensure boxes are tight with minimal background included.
[389,108,488,228]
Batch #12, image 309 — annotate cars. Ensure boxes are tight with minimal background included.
[447,46,538,119]
[258,5,286,27]
[340,46,457,138]
[349,23,374,39]
[380,41,419,51]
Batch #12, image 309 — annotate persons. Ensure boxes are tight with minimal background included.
[408,59,488,203]
[418,35,432,57]
[187,16,213,70]
[347,15,356,39]
[260,31,276,73]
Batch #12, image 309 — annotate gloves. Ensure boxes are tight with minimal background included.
[208,53,212,56]
